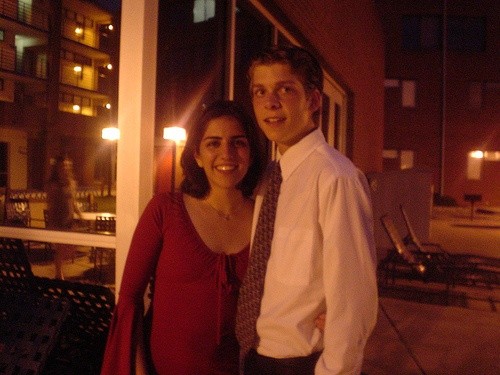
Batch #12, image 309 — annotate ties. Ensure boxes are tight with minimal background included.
[235,158,282,365]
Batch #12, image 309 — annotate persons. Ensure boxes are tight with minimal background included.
[234,44,379,375]
[42,154,84,282]
[99,99,267,374]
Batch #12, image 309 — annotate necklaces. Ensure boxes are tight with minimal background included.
[201,194,243,222]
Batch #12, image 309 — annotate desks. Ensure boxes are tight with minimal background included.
[72,212,116,262]
